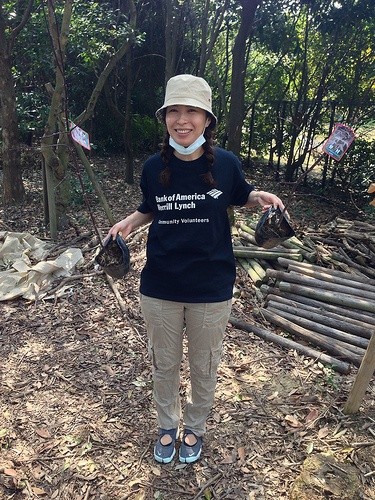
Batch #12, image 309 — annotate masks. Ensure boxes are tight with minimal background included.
[169,128,206,155]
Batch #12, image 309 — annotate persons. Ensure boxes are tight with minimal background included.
[104,73,291,463]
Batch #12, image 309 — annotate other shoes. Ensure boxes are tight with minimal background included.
[154,426,179,463]
[179,429,203,463]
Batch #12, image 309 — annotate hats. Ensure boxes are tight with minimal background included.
[155,74,217,130]
